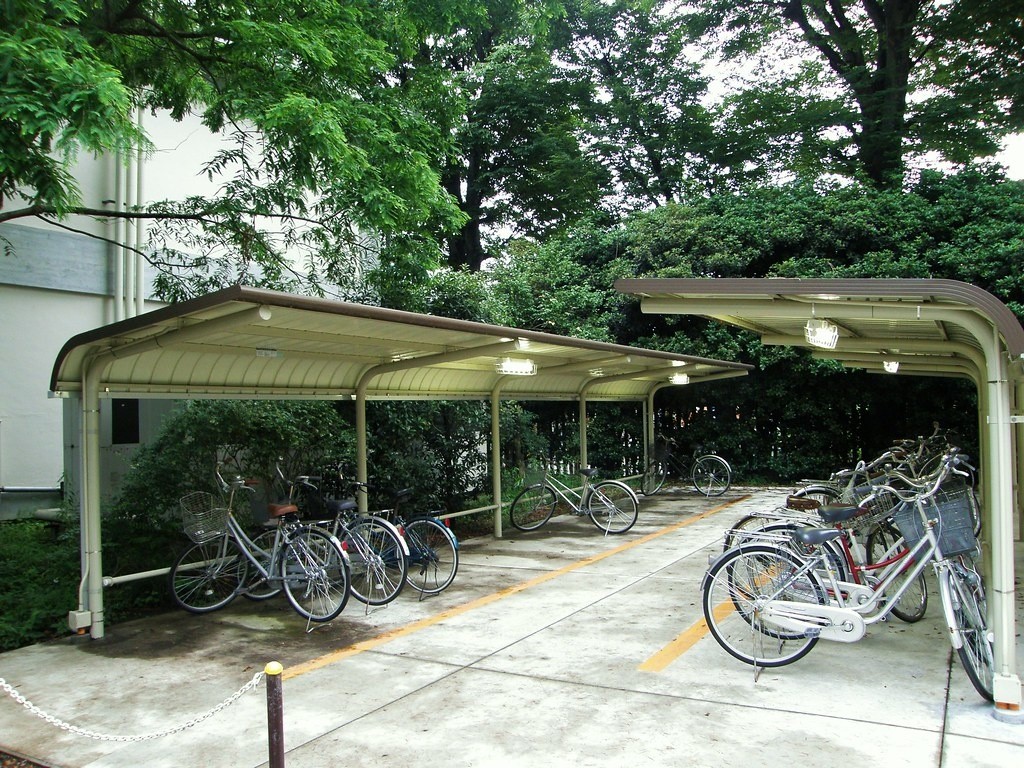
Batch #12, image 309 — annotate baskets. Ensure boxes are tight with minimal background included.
[891,496,975,564]
[521,459,545,485]
[836,474,894,524]
[649,443,668,461]
[180,491,227,543]
[923,476,967,505]
[248,484,280,524]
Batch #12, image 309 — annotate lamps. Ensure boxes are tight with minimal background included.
[494,357,537,375]
[668,373,690,384]
[803,319,839,349]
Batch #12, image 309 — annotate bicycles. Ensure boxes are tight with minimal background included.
[701,415,995,703]
[169,458,352,634]
[640,431,732,498]
[237,455,461,616]
[510,447,641,537]
